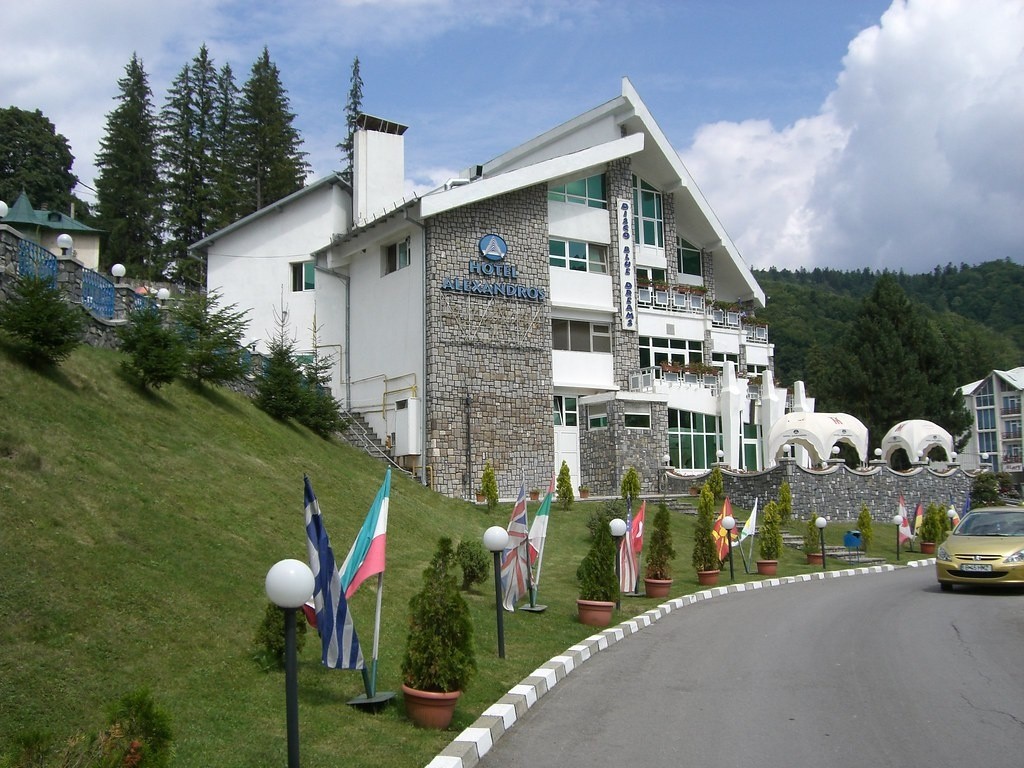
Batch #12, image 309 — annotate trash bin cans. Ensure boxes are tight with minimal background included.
[844,530,861,547]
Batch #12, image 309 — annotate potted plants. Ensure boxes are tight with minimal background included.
[693,481,721,583]
[662,361,782,387]
[400,537,477,728]
[702,299,740,312]
[921,504,941,554]
[477,492,486,502]
[804,512,823,564]
[642,503,675,596]
[579,484,590,498]
[636,278,704,294]
[530,488,540,500]
[755,501,783,574]
[575,510,620,624]
[742,317,768,326]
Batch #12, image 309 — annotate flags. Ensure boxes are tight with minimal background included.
[731,498,758,547]
[529,482,553,566]
[711,496,738,561]
[911,498,923,540]
[898,495,911,545]
[500,481,531,612]
[619,494,645,592]
[303,477,367,670]
[303,471,391,627]
[950,498,960,526]
[960,494,970,520]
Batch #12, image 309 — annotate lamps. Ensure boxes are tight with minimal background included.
[783,443,790,457]
[0,200,9,223]
[982,452,989,462]
[874,448,883,460]
[664,455,671,466]
[57,234,72,255]
[716,450,724,462]
[157,288,170,306]
[917,449,924,461]
[112,263,126,283]
[832,446,841,459]
[950,452,957,462]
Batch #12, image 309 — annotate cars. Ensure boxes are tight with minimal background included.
[935,504,1024,591]
